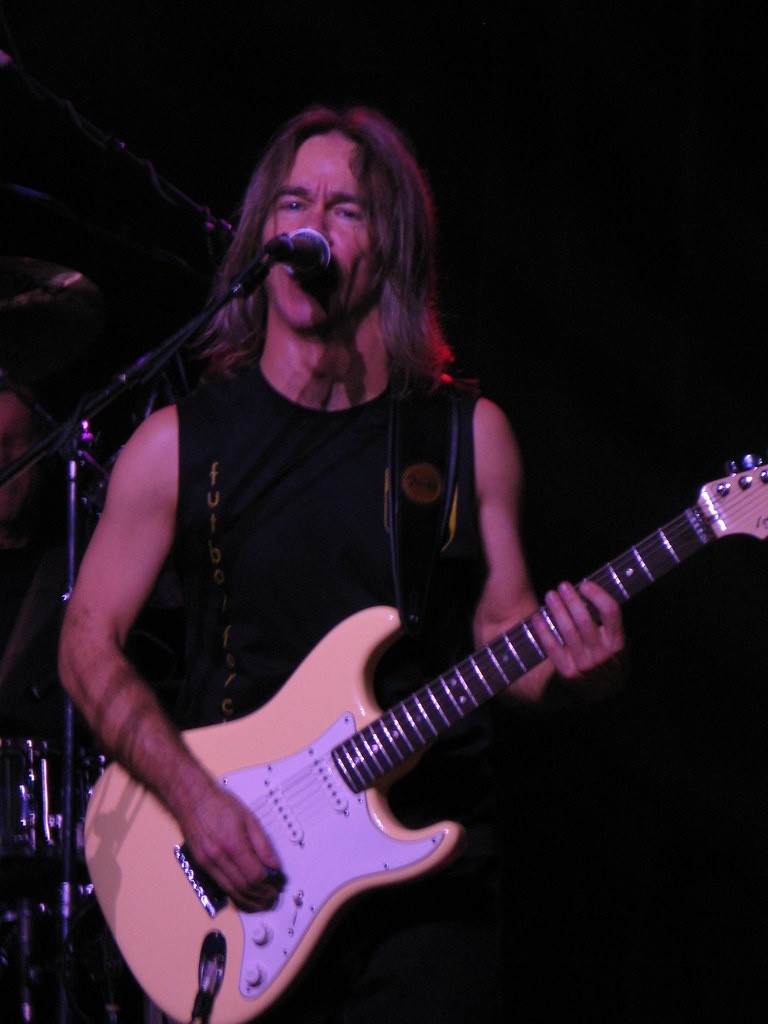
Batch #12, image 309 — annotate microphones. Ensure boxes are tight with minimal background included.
[266,229,332,280]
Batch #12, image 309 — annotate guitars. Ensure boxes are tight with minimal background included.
[82,443,768,1024]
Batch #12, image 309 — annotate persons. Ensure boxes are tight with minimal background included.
[54,103,627,1024]
[0,368,69,736]
